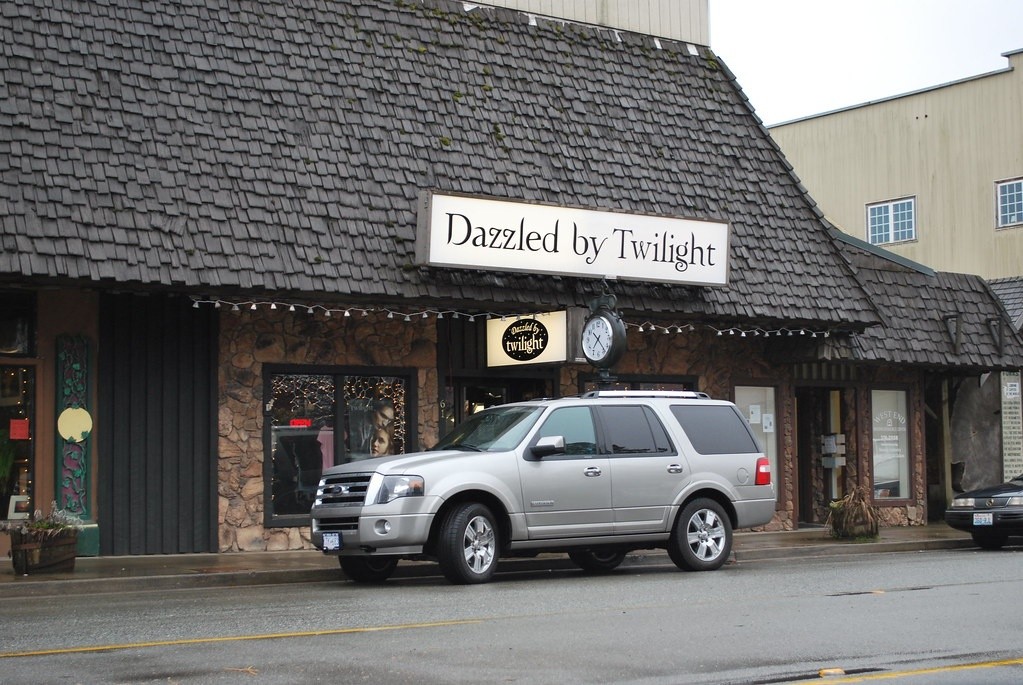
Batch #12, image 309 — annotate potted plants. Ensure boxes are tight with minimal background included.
[823,484,880,536]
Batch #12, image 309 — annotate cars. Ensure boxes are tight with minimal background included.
[945,472,1023,550]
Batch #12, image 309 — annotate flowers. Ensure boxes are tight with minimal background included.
[0,499,85,535]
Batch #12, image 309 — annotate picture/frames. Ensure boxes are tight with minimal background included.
[7,495,29,519]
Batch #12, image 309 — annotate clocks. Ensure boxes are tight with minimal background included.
[581,311,626,369]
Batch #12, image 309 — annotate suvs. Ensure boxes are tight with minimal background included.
[308,387,777,584]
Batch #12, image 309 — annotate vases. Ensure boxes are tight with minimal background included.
[10,529,76,573]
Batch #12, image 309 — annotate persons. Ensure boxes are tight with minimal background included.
[349,400,395,459]
